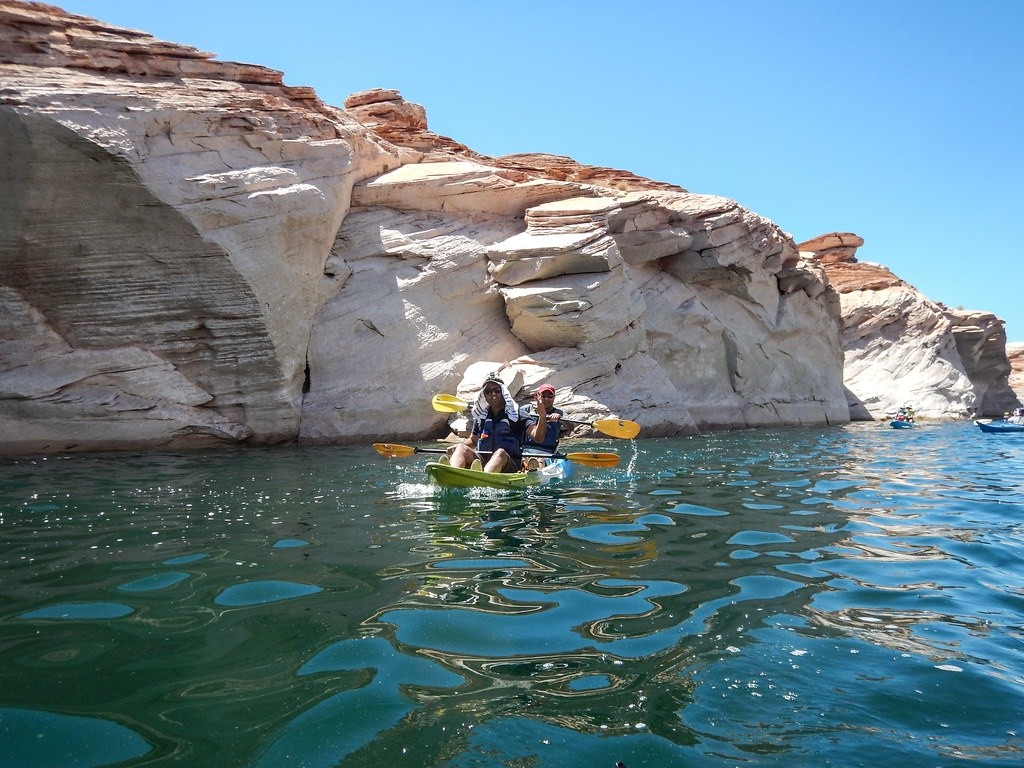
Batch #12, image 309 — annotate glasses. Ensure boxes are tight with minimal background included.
[485,387,503,395]
[540,393,554,399]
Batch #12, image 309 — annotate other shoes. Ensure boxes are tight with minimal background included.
[438,455,450,465]
[527,457,539,471]
[470,459,484,471]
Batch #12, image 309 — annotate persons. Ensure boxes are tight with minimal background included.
[895,405,916,423]
[520,384,575,471]
[438,374,547,473]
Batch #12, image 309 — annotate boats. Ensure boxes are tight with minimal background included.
[889,420,918,428]
[975,419,1024,433]
[421,457,577,489]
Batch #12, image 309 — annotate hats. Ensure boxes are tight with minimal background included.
[482,370,504,387]
[906,407,912,409]
[537,384,555,398]
[900,406,906,409]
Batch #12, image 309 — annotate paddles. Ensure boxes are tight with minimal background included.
[431,393,641,441]
[371,441,621,469]
